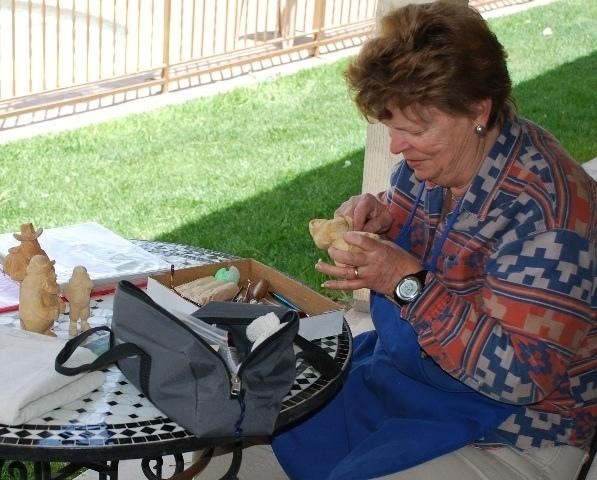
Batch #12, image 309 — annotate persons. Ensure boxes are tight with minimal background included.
[194,0,597,479]
[2,224,93,338]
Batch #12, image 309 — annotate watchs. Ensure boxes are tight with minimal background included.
[392,269,429,308]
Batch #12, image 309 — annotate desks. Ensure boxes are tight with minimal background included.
[0,238,353,480]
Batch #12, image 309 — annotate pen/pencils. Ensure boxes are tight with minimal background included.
[269,291,305,312]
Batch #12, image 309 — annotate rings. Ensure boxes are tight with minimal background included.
[353,267,359,280]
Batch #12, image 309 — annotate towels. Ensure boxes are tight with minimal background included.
[0,327,106,427]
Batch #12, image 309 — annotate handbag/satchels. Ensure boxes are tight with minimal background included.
[53,275,343,442]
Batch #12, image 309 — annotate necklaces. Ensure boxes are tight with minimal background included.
[450,191,461,204]
